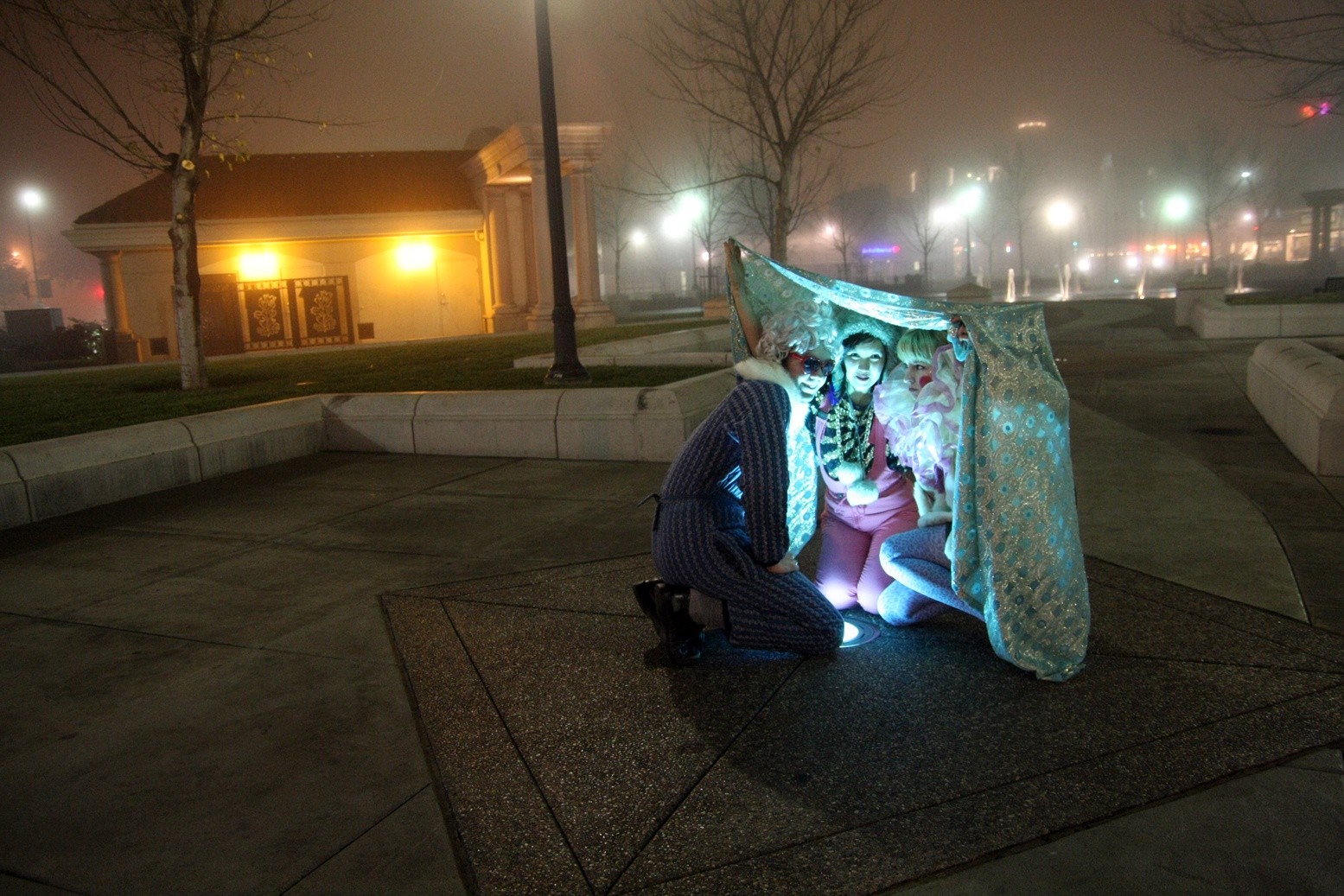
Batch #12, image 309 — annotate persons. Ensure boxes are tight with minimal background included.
[876,318,985,627]
[726,239,921,615]
[638,307,841,666]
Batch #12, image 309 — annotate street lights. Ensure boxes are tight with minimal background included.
[677,193,705,292]
[18,190,46,308]
[955,186,983,283]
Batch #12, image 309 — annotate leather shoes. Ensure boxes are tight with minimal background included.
[654,585,705,669]
[631,577,663,632]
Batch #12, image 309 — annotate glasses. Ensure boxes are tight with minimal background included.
[786,352,835,377]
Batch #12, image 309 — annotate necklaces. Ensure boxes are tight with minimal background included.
[833,402,875,466]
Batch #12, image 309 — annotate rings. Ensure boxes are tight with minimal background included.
[795,560,798,565]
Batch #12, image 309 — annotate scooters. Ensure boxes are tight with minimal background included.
[62,315,111,361]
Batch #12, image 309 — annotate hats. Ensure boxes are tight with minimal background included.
[830,308,902,507]
